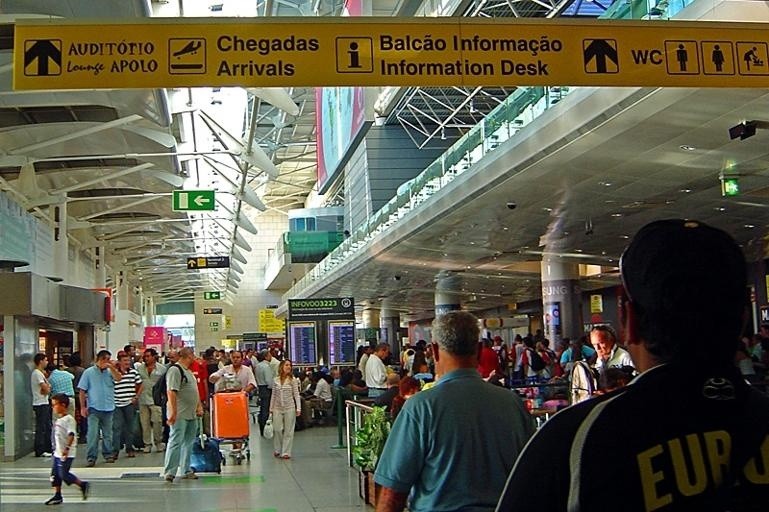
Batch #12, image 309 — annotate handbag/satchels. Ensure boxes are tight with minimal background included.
[263,412,273,440]
[215,373,242,391]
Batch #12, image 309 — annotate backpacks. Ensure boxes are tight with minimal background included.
[152,364,188,407]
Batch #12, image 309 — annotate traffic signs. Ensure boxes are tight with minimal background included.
[12,17,769,88]
[203,291,222,300]
[172,188,216,212]
[186,255,230,270]
[204,308,222,314]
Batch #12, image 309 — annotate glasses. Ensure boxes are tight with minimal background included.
[50,402,61,408]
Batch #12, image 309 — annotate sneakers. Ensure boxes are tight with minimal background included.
[143,447,152,453]
[41,452,51,457]
[156,445,163,451]
[165,473,173,481]
[184,473,198,479]
[45,496,63,506]
[104,457,114,462]
[284,455,288,458]
[114,455,119,459]
[128,451,136,458]
[81,481,90,500]
[274,451,279,455]
[85,460,96,468]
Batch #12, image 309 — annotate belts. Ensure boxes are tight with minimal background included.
[68,394,75,399]
[368,386,389,391]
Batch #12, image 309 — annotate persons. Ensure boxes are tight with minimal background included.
[201,346,232,372]
[138,348,167,452]
[294,363,367,432]
[387,376,421,421]
[79,350,122,467]
[558,323,637,406]
[254,350,276,436]
[65,352,87,442]
[31,353,53,458]
[365,342,390,397]
[209,350,258,460]
[493,221,769,512]
[269,359,302,459]
[242,351,254,400]
[44,363,76,420]
[400,340,433,381]
[123,343,136,369]
[269,349,281,377]
[133,355,145,368]
[373,374,402,412]
[733,322,769,385]
[187,347,210,437]
[358,346,373,379]
[383,345,394,366]
[163,348,204,483]
[112,351,145,460]
[164,350,179,367]
[42,393,89,507]
[254,351,258,356]
[477,323,557,401]
[370,312,538,511]
[245,348,259,369]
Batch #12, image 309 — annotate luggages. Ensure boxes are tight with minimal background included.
[214,391,249,439]
[190,415,224,474]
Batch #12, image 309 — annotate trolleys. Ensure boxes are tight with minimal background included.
[208,388,251,464]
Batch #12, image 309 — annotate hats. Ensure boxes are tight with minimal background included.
[618,216,750,363]
[493,336,504,342]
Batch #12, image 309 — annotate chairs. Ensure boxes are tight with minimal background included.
[315,396,336,424]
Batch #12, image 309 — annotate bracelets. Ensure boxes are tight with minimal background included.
[66,446,70,450]
